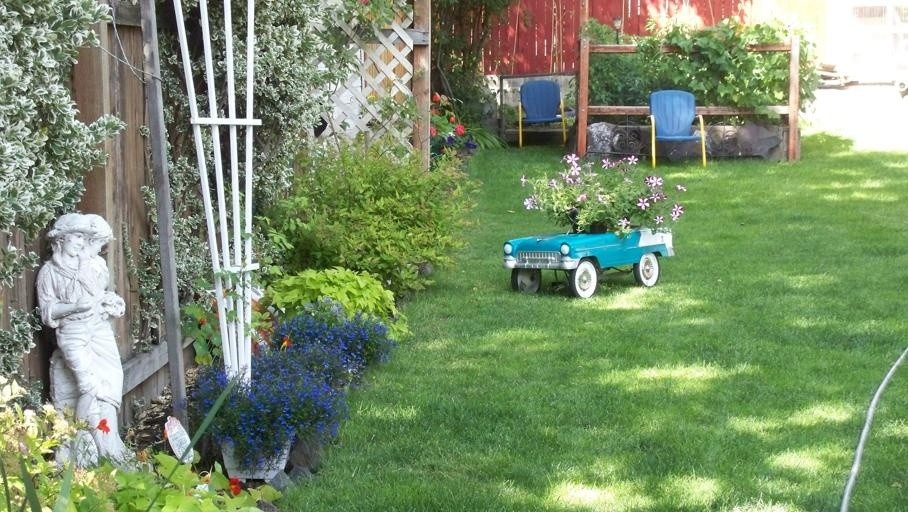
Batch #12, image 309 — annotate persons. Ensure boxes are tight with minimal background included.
[47,214,117,461]
[36,212,132,470]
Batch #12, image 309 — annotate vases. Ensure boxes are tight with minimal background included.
[216,436,298,484]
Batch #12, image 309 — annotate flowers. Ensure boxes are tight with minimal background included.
[192,304,396,471]
[519,153,686,241]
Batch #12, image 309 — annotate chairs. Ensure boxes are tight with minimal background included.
[648,91,710,168]
[518,80,567,148]
